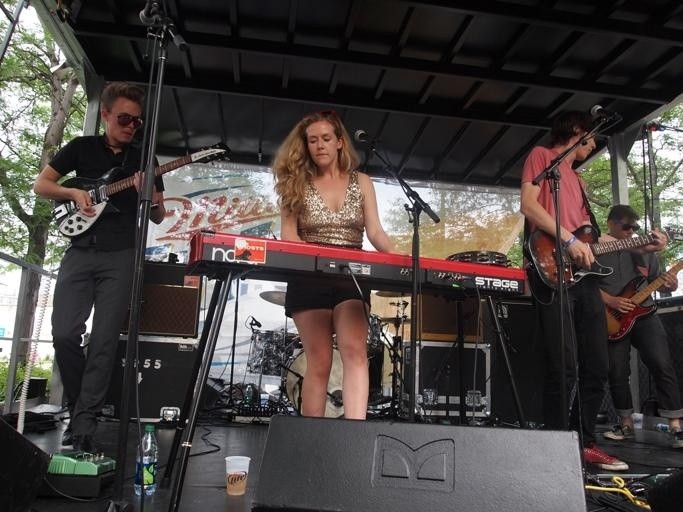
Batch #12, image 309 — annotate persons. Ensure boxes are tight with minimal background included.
[520,116,668,471]
[33,82,164,454]
[596,205,683,449]
[272,110,412,420]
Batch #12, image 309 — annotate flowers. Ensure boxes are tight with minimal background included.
[252,316,261,328]
[590,105,623,120]
[139,1,155,27]
[353,129,379,144]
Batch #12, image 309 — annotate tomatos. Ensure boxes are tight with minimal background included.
[186,229,527,299]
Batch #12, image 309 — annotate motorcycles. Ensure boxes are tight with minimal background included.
[532,123,650,490]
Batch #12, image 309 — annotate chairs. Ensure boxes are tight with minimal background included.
[671,427,683,448]
[581,443,629,471]
[603,424,635,440]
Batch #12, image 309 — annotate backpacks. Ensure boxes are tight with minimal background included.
[246,330,295,376]
[282,346,345,418]
[445,251,510,265]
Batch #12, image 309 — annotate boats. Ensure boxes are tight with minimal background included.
[411,287,483,343]
[252,413,587,512]
[202,378,225,411]
[119,282,199,337]
[143,261,203,303]
[1,418,53,511]
[477,300,545,428]
[569,296,683,423]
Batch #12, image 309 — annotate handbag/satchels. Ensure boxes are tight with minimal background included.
[562,236,577,250]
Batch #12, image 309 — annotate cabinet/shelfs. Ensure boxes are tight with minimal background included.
[133,423,159,496]
[653,422,670,433]
[243,384,254,408]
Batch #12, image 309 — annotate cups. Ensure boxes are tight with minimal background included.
[619,221,640,231]
[116,112,144,129]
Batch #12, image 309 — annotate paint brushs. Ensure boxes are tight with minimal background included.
[231,415,271,425]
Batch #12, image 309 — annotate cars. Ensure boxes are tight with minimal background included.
[225,455,252,498]
[631,413,643,429]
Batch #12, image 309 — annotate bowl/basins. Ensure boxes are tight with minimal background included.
[109,144,123,147]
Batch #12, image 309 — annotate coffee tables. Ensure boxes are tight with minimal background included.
[258,292,286,306]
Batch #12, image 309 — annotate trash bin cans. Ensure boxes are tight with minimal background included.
[151,200,160,210]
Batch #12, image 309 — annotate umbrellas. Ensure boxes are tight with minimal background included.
[62,424,94,452]
